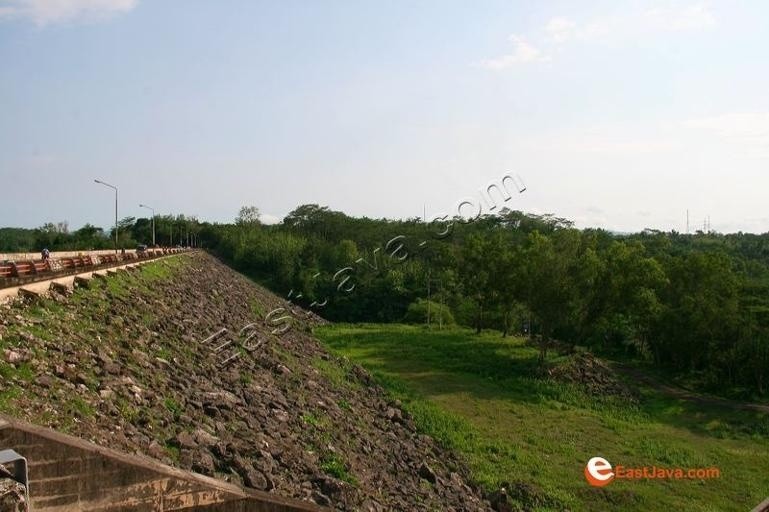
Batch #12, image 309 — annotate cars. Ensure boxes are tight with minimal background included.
[137,244,147,250]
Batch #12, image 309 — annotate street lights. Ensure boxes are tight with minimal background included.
[139,204,155,246]
[94,178,118,250]
